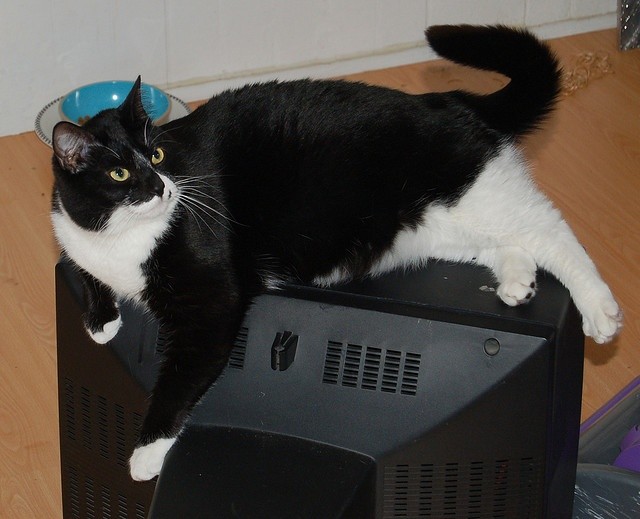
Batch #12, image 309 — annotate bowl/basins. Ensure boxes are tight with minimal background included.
[58,80,171,127]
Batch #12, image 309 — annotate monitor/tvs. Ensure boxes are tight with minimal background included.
[54,251,585,519]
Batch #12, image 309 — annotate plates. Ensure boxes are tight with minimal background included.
[33,93,192,147]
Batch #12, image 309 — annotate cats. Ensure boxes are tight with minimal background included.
[49,24,620,481]
